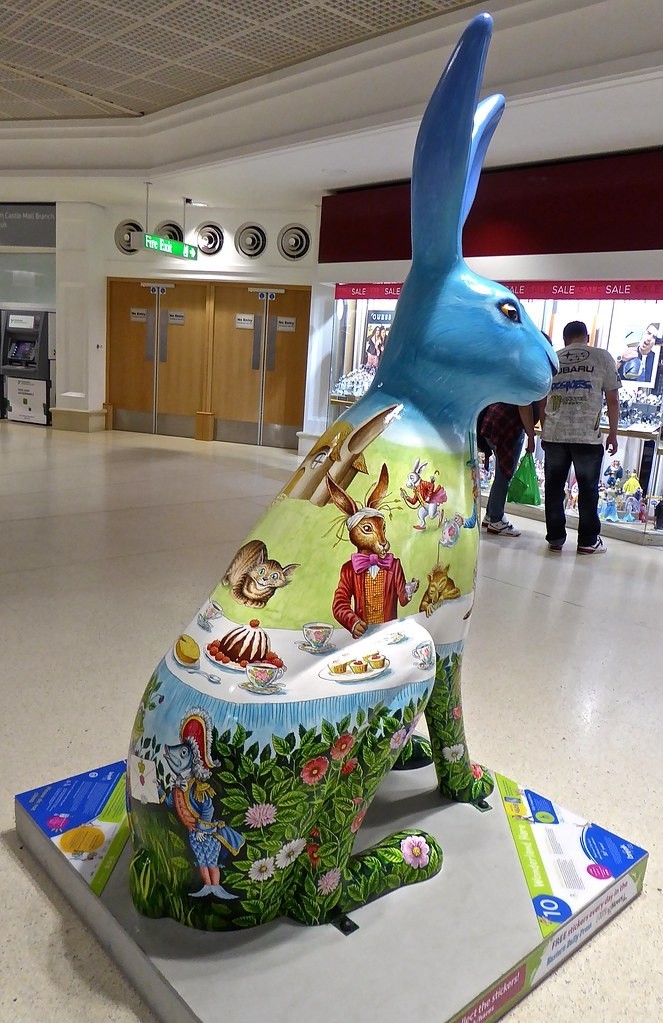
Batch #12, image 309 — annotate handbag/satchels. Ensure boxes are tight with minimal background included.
[506,452,541,506]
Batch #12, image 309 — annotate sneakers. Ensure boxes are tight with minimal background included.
[487,515,521,537]
[577,535,606,554]
[482,513,491,527]
[548,543,562,552]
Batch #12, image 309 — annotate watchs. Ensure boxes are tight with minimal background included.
[617,355,623,363]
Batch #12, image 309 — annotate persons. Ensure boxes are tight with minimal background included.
[597,460,648,523]
[480,321,623,555]
[616,323,659,382]
[366,326,388,367]
